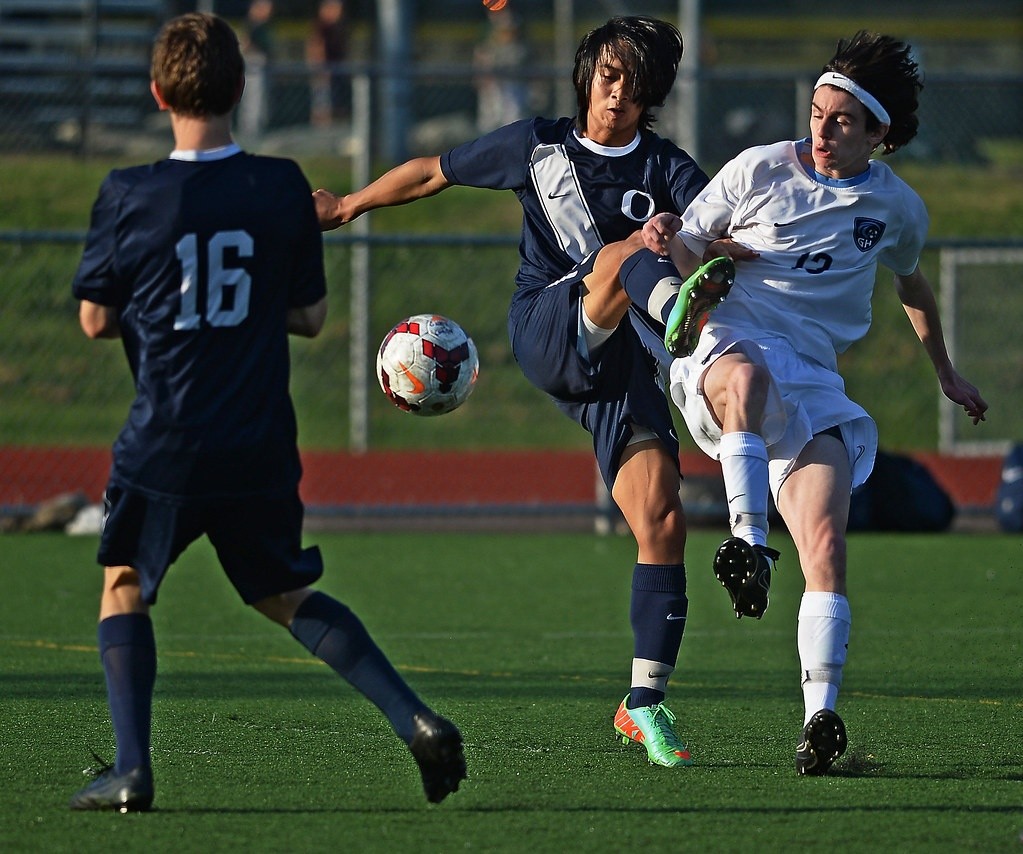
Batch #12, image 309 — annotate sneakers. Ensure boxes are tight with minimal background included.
[795,708,848,776]
[409,712,466,804]
[712,536,772,619]
[663,255,736,358]
[69,764,153,810]
[613,693,693,768]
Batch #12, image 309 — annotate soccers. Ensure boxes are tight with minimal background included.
[377,314,477,417]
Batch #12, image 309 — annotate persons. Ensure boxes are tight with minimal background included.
[307,17,759,773]
[641,29,993,786]
[68,14,467,815]
[224,2,563,163]
